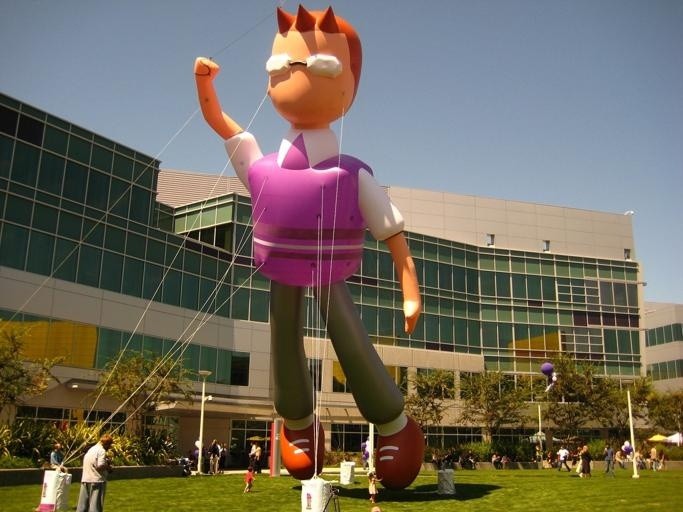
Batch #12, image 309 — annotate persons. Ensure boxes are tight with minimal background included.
[603,443,616,479]
[543,446,591,478]
[363,435,369,470]
[50,444,68,473]
[615,449,625,468]
[76,434,113,512]
[432,449,511,469]
[248,441,262,473]
[242,466,255,493]
[195,3,425,490]
[208,439,223,474]
[635,444,665,471]
[368,473,383,503]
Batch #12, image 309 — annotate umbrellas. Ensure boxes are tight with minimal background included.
[648,432,683,442]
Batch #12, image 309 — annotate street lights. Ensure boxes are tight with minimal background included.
[622,379,640,479]
[534,395,545,469]
[197,370,213,475]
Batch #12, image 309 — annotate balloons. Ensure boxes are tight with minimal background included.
[621,441,632,454]
[361,443,369,460]
